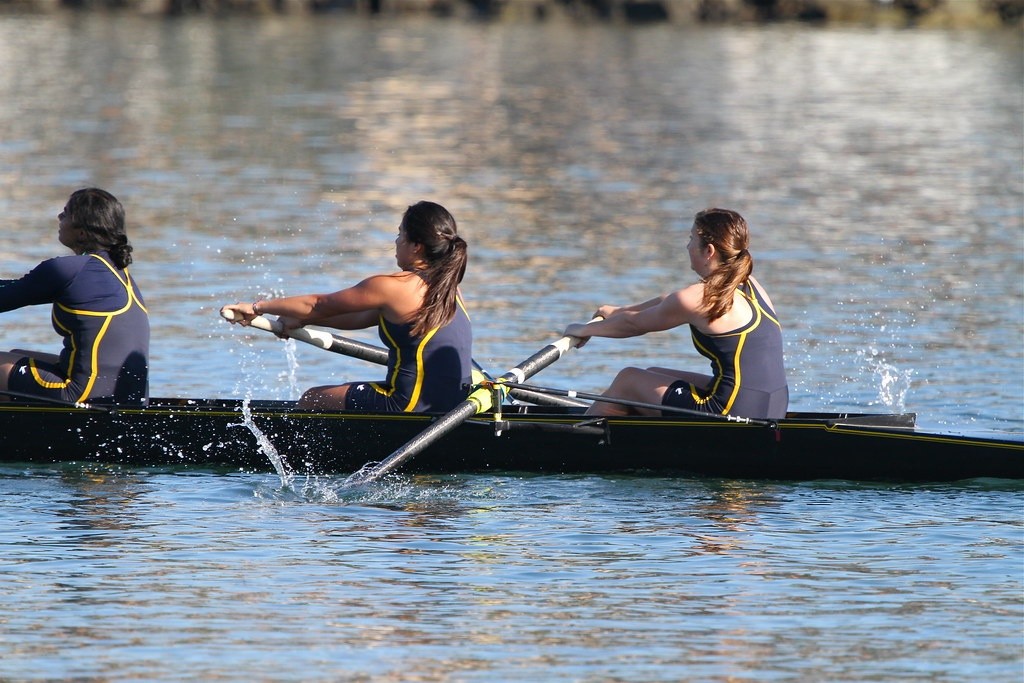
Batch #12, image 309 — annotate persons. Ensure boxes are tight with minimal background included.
[562,208,790,419]
[0,188,151,407]
[220,201,473,416]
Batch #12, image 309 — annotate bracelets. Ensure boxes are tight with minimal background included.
[253,303,263,316]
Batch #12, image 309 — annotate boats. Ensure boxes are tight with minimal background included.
[0,396,1024,480]
